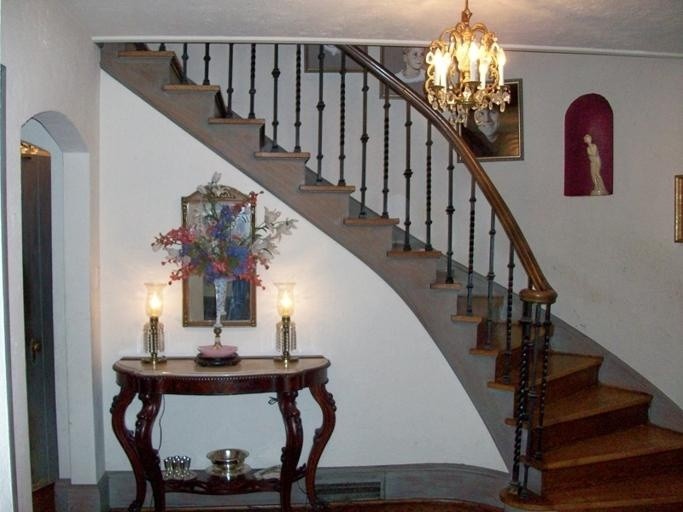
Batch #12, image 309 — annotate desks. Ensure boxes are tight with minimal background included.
[110,357,338,510]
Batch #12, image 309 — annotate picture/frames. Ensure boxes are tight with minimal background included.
[380,46,425,99]
[181,185,256,325]
[305,44,365,73]
[457,77,524,162]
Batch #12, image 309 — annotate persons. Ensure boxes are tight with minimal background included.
[465,104,511,156]
[394,47,425,95]
[584,132,605,189]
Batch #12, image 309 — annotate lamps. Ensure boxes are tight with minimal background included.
[273,281,299,366]
[141,281,168,368]
[425,0,511,127]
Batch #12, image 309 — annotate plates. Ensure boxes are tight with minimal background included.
[205,462,251,482]
[198,344,238,359]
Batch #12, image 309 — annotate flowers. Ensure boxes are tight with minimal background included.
[151,172,299,291]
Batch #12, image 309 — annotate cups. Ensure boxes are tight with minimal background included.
[164,456,190,481]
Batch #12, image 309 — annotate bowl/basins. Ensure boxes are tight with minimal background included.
[207,449,250,471]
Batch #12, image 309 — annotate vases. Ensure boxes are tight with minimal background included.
[207,280,233,317]
